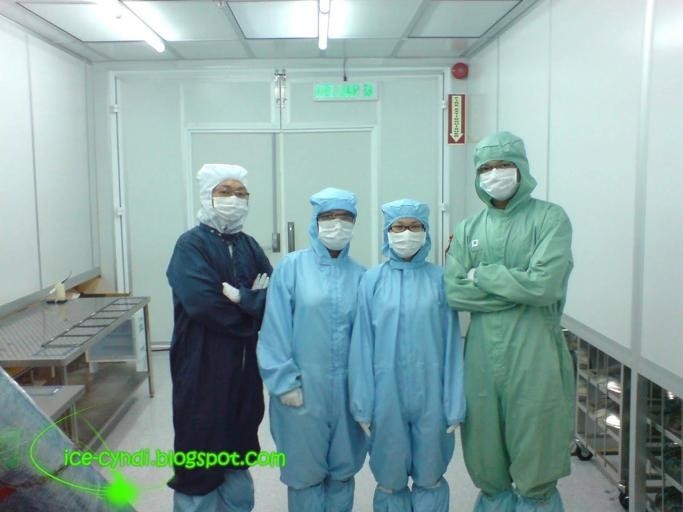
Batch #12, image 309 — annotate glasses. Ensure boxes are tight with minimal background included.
[317,212,353,220]
[218,189,249,198]
[390,225,423,232]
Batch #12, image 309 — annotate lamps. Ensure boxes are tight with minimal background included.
[93,0,169,57]
[318,0,332,53]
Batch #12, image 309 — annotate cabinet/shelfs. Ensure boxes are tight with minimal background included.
[560,320,683,512]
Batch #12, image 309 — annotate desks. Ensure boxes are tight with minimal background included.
[0,296,158,452]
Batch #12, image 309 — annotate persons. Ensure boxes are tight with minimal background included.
[255,185,367,509]
[443,129,573,510]
[347,200,468,510]
[165,163,275,511]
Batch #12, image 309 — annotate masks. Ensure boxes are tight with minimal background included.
[478,168,519,200]
[317,218,353,251]
[387,230,426,258]
[213,195,247,224]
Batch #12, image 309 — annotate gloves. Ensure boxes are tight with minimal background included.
[359,421,371,436]
[280,388,303,407]
[222,282,241,304]
[447,423,459,434]
[251,272,269,290]
